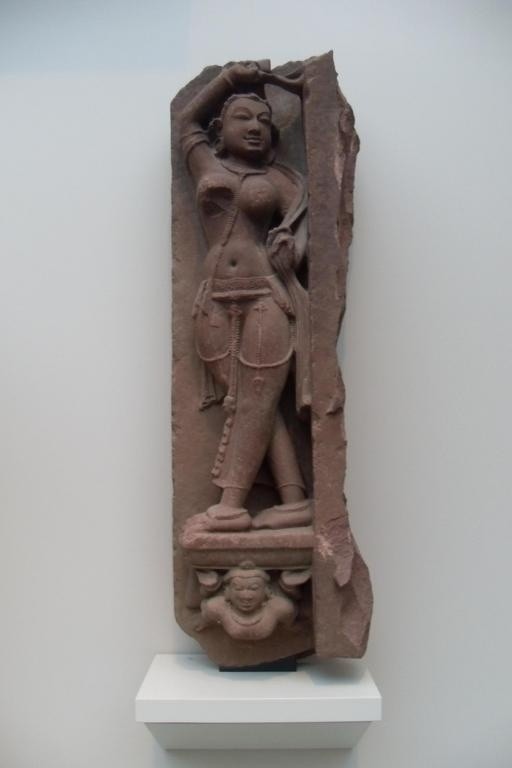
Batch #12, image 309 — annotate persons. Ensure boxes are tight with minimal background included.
[180,65,312,531]
[185,558,311,643]
